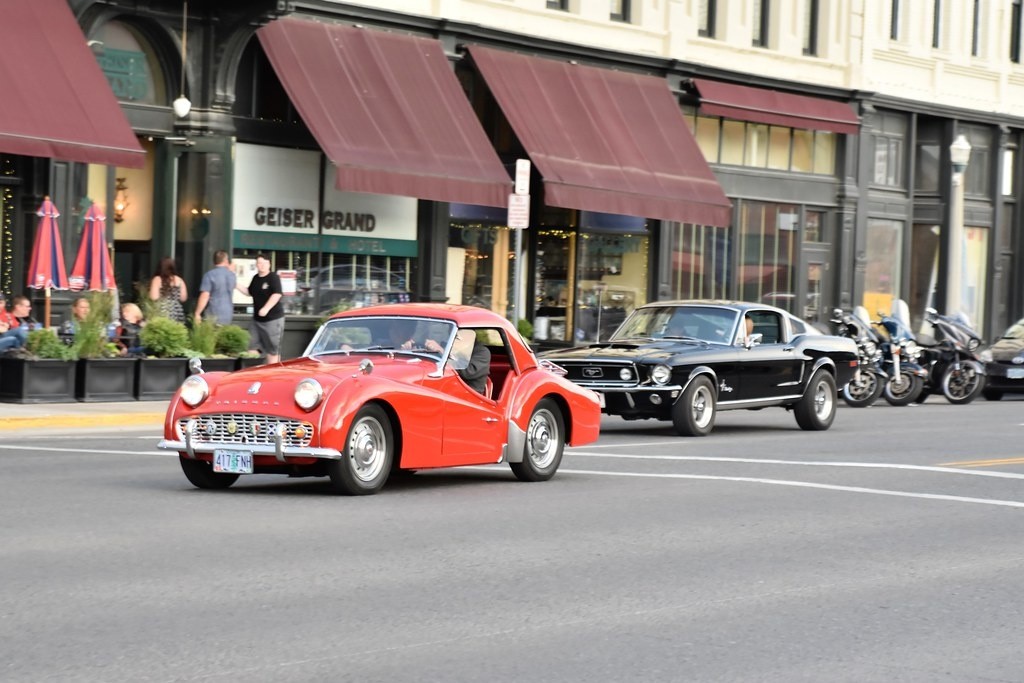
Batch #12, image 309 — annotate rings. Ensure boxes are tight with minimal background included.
[401,344,404,346]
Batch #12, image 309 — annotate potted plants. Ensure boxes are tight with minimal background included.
[0,272,265,405]
[517,318,540,354]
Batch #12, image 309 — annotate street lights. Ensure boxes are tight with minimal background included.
[942,133,971,316]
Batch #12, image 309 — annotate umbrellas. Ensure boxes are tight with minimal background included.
[28,197,69,330]
[64,198,119,315]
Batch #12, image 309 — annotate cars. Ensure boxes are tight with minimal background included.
[980,315,1024,402]
[533,297,862,438]
[298,261,411,316]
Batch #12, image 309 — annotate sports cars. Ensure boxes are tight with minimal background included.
[155,299,603,497]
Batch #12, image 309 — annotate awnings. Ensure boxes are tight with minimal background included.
[733,259,786,282]
[673,249,706,277]
[459,40,735,229]
[258,14,515,211]
[1,0,149,169]
[693,79,861,135]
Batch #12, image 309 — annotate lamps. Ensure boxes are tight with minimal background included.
[173,0,192,117]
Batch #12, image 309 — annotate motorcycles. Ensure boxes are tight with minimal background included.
[811,292,987,407]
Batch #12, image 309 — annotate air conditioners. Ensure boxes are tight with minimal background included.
[113,178,130,223]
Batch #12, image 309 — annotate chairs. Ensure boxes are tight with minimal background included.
[483,377,494,399]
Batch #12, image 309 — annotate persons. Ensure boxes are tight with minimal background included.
[0,293,33,353]
[400,323,491,395]
[536,294,569,317]
[235,252,286,365]
[535,288,547,302]
[340,318,416,352]
[194,250,238,329]
[109,300,160,357]
[148,254,188,326]
[60,296,107,345]
